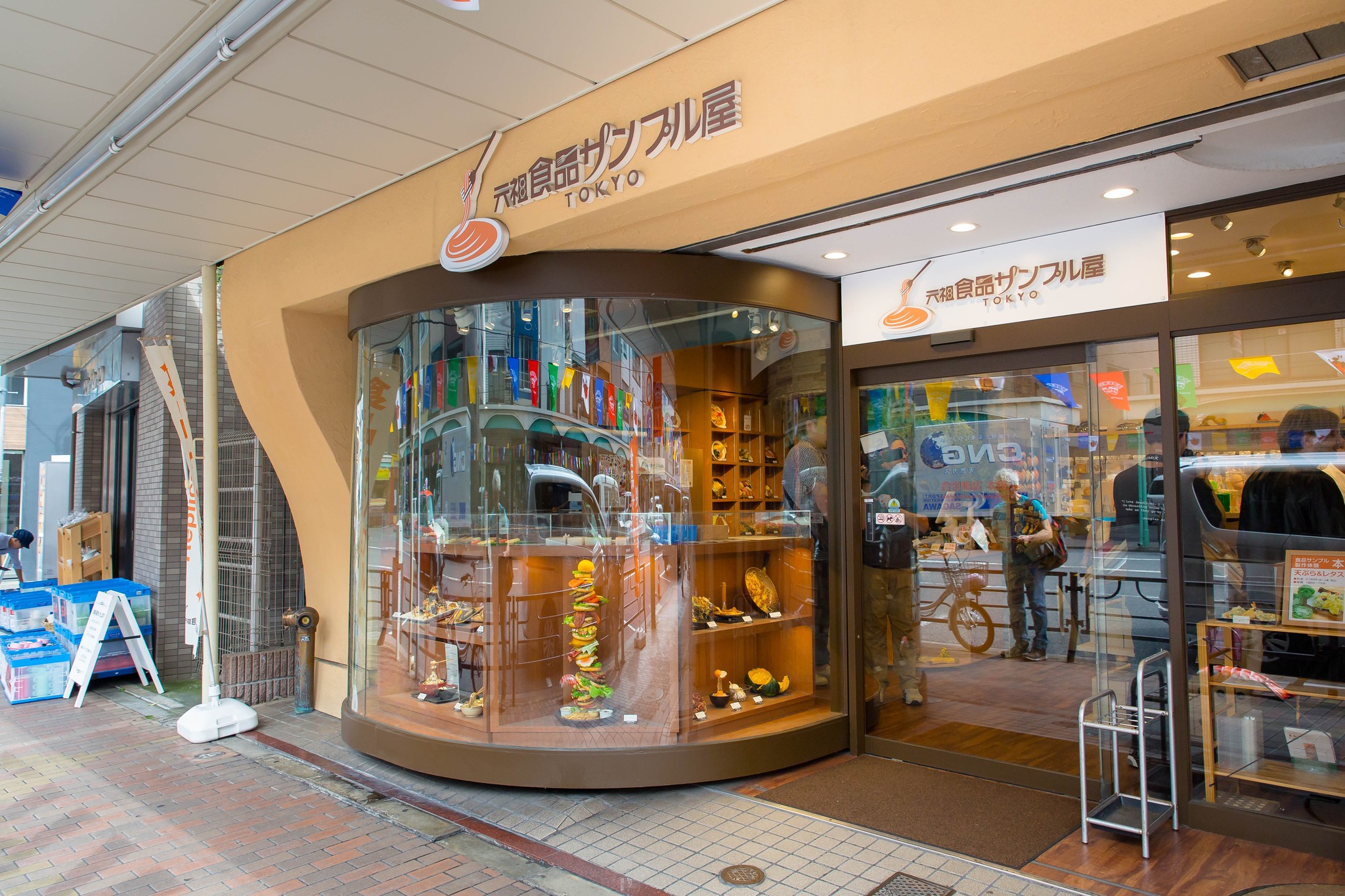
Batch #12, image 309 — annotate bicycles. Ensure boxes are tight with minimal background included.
[914,550,995,655]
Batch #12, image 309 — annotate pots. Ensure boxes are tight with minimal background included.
[741,552,778,614]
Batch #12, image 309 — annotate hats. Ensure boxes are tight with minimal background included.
[887,434,902,448]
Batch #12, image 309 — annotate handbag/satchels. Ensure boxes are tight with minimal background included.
[1020,498,1068,572]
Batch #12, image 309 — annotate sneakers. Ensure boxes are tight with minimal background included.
[880,689,883,702]
[1000,643,1029,658]
[902,688,923,705]
[815,665,830,681]
[815,674,828,686]
[1023,648,1048,661]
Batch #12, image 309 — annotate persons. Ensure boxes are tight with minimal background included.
[0,529,33,586]
[1237,401,1345,815]
[857,432,929,706]
[1108,405,1227,776]
[987,466,1073,663]
[413,476,436,579]
[781,402,835,688]
[485,467,513,604]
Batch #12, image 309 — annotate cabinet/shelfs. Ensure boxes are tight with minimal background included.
[380,385,1344,824]
[57,511,112,585]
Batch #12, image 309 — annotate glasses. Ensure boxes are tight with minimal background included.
[901,450,908,457]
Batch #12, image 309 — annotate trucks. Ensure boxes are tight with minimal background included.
[419,463,609,544]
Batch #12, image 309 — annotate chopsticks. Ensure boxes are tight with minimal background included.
[475,686,483,696]
[447,600,464,626]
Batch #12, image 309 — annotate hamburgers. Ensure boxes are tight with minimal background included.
[562,559,617,720]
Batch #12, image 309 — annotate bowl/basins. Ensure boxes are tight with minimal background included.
[418,678,448,698]
[709,691,732,708]
[435,684,458,700]
[599,710,613,719]
[460,703,484,717]
[560,706,573,717]
[711,403,730,536]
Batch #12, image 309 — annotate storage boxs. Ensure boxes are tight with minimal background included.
[0,578,152,704]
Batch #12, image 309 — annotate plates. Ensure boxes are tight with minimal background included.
[713,610,744,617]
[443,606,484,625]
[555,707,617,727]
[1216,618,1281,625]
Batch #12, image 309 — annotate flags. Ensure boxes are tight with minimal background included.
[390,356,684,480]
[856,341,1345,408]
